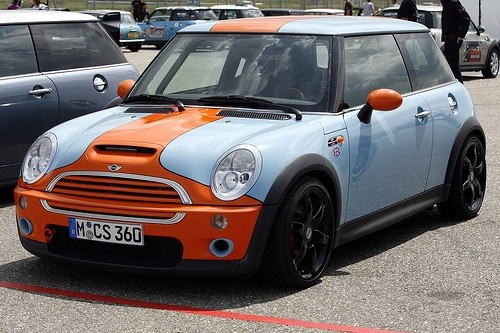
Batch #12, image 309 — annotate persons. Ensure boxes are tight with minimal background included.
[362,0,376,16]
[345,0,352,16]
[8,0,198,22]
[397,0,418,22]
[440,0,471,84]
[390,0,400,7]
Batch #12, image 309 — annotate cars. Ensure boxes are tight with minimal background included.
[0,9,149,187]
[135,6,218,49]
[209,5,265,51]
[379,6,500,78]
[261,9,306,16]
[306,8,345,16]
[13,15,487,289]
[78,10,145,52]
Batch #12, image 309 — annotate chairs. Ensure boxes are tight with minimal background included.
[263,39,328,103]
